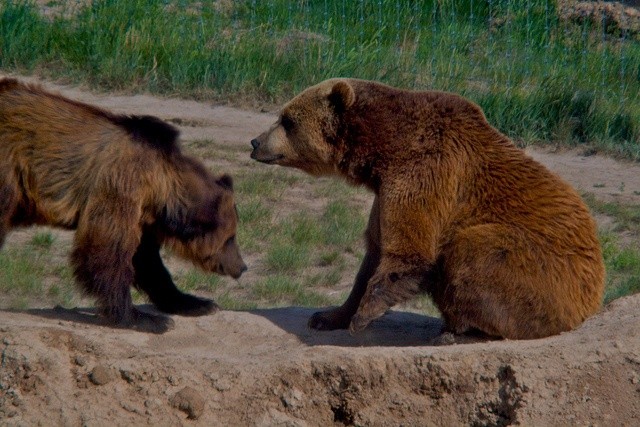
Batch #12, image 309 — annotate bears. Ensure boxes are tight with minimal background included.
[0,74,249,336]
[250,78,606,340]
